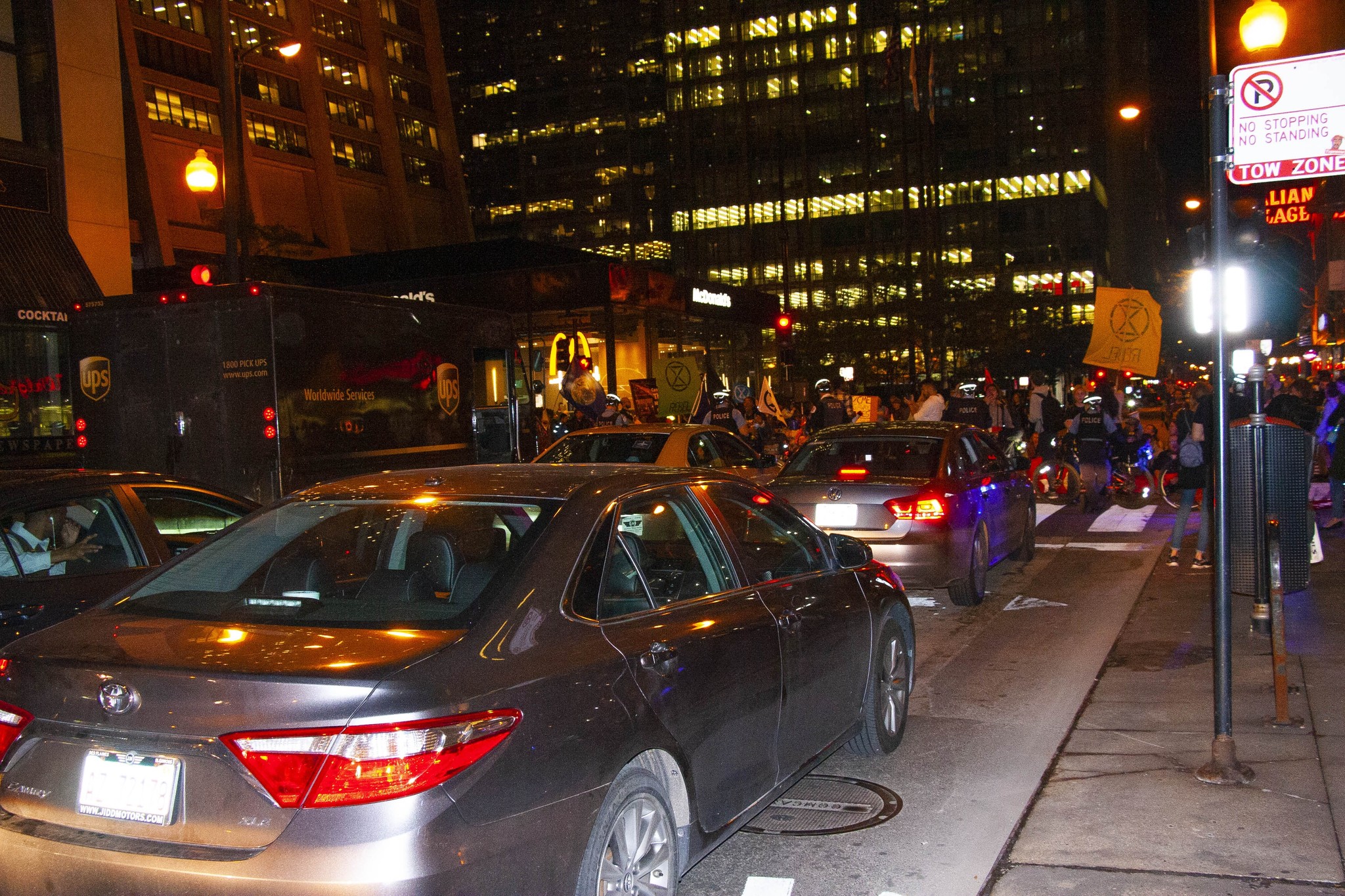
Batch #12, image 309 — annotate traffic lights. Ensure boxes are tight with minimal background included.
[189,260,227,287]
[579,356,592,370]
[779,317,792,336]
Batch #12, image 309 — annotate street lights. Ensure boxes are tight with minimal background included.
[186,0,302,279]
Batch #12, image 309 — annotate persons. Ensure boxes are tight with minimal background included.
[547,378,1344,570]
[0,500,103,577]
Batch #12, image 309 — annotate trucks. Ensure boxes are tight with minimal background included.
[67,278,543,505]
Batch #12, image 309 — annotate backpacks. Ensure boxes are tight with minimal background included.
[1178,433,1203,468]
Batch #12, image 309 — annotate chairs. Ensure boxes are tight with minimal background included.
[255,529,661,622]
[71,507,129,573]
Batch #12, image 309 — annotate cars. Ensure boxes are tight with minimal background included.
[1,460,916,896]
[745,420,1037,606]
[1,468,263,648]
[529,421,783,486]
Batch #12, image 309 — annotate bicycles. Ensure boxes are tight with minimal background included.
[1028,434,1156,509]
[1156,454,1211,514]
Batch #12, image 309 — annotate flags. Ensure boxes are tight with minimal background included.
[1082,286,1163,377]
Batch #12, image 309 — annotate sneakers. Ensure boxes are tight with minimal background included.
[1165,554,1180,567]
[1191,558,1213,568]
